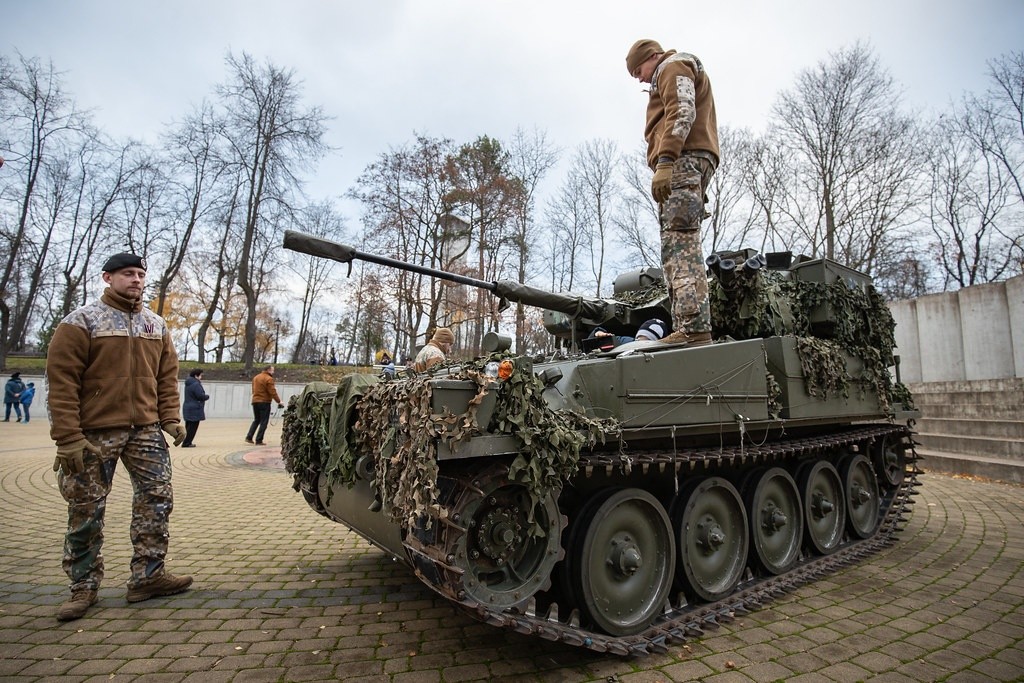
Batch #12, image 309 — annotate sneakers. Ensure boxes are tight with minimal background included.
[657,330,713,347]
[57,589,98,619]
[245,438,254,443]
[256,441,267,445]
[127,571,194,601]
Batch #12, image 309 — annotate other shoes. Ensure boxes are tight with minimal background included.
[16,419,21,421]
[0,418,8,421]
[189,443,196,446]
[21,418,27,423]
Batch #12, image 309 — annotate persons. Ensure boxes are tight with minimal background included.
[0,372,24,422]
[19,382,35,423]
[412,328,455,372]
[181,369,209,447]
[47,253,195,622]
[588,319,666,347]
[246,365,283,446]
[625,39,719,349]
[380,353,412,381]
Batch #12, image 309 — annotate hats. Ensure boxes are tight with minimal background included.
[102,252,147,271]
[433,327,454,343]
[626,38,665,76]
[635,317,667,340]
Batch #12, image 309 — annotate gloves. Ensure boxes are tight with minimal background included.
[651,162,673,203]
[164,422,187,446]
[52,438,102,475]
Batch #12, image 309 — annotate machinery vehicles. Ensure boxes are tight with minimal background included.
[279,231,923,658]
